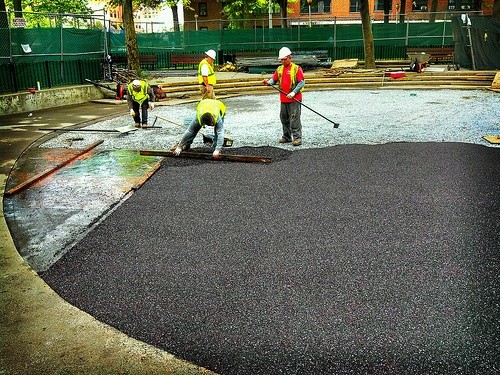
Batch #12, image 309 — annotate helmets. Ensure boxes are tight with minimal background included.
[278,47,292,59]
[205,50,216,60]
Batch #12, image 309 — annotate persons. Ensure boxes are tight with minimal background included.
[197,49,217,100]
[172,98,227,160]
[126,80,155,129]
[263,46,305,146]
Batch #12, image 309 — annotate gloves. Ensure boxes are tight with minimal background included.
[173,146,182,156]
[262,79,270,85]
[286,92,295,99]
[130,109,135,116]
[213,150,219,159]
[205,86,211,93]
[150,102,154,110]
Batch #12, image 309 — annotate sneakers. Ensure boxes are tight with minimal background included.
[293,140,302,146]
[135,123,140,127]
[280,138,287,143]
[171,143,186,150]
[142,124,147,128]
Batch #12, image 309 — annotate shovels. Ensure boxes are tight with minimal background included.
[267,82,340,128]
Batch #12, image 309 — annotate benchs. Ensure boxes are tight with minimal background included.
[171,54,205,70]
[115,56,158,71]
[405,48,455,65]
